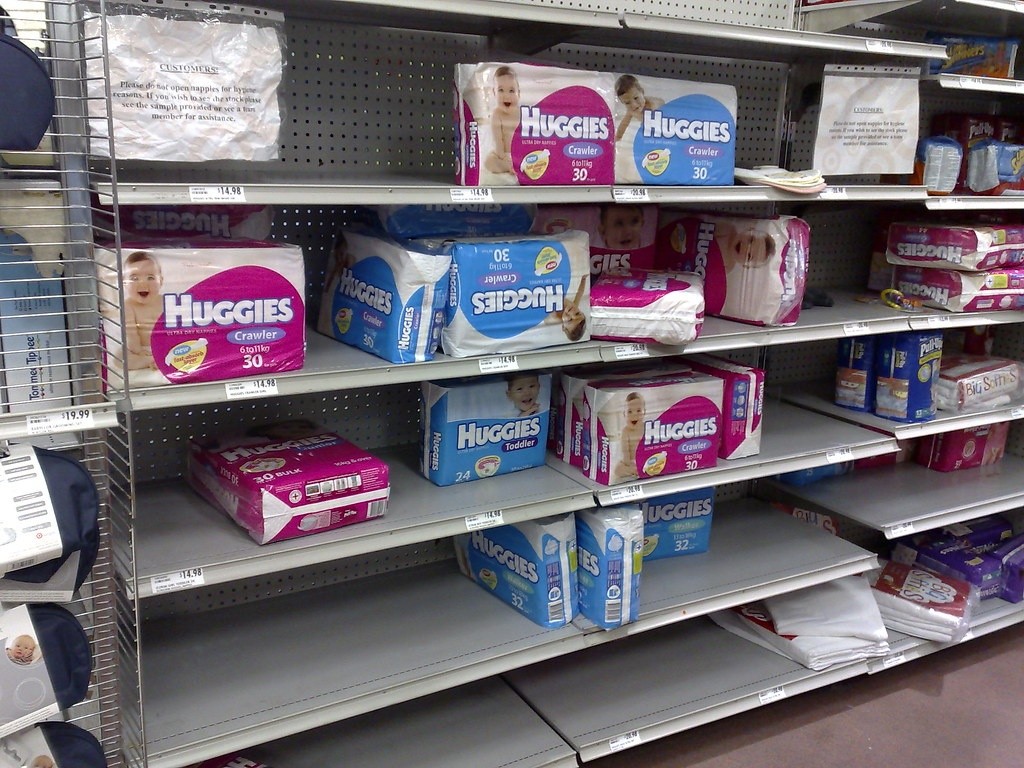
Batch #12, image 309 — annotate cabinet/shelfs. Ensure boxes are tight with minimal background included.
[801,0,1024,676]
[79,1,602,768]
[501,2,951,762]
[1,1,126,767]
